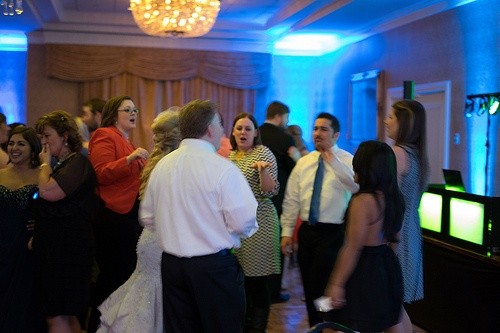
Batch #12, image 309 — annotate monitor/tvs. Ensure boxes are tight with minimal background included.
[416,185,494,258]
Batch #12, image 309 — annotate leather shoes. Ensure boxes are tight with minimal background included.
[271,294,289,304]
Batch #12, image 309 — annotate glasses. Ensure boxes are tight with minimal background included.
[116,107,138,113]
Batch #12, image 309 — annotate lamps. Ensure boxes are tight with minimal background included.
[129,0,221,39]
[0,0,24,16]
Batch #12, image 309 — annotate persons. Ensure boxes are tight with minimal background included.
[226,112,279,333]
[95,105,184,332]
[0,125,49,333]
[0,113,12,169]
[324,141,405,333]
[35,110,104,333]
[87,96,150,290]
[140,98,258,333]
[82,98,108,131]
[384,101,430,333]
[281,123,311,290]
[282,112,359,333]
[257,101,302,304]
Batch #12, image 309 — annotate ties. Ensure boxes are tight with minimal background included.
[308,156,325,226]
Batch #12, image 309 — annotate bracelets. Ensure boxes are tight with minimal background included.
[39,162,51,170]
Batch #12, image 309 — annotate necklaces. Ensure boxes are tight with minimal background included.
[51,149,78,171]
